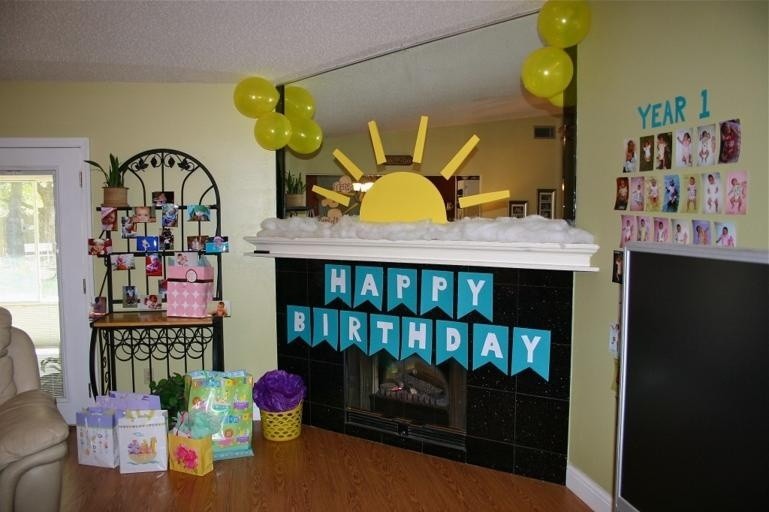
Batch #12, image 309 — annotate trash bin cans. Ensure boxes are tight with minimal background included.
[259,382,303,442]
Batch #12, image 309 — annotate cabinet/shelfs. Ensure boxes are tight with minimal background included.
[305,174,480,220]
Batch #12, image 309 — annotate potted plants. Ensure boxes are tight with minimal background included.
[83,153,129,207]
[284,170,308,207]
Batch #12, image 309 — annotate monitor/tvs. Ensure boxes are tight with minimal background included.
[614,240,769,512]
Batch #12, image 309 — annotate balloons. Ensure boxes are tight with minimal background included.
[546,92,564,107]
[288,119,323,155]
[232,77,280,119]
[521,47,574,98]
[283,86,315,122]
[252,112,293,151]
[536,1,592,48]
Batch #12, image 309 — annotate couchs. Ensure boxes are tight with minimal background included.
[0,305,71,512]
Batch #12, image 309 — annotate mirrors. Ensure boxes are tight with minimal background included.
[275,8,577,221]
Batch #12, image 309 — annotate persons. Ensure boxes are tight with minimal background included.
[87,191,232,319]
[613,117,748,282]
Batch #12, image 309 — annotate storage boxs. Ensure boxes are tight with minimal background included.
[166,265,215,318]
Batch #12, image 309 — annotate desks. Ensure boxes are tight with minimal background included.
[89,310,224,400]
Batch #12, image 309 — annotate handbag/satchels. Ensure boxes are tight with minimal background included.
[76,406,120,469]
[165,262,213,317]
[116,408,168,474]
[169,427,215,476]
[97,390,161,410]
[184,368,255,460]
